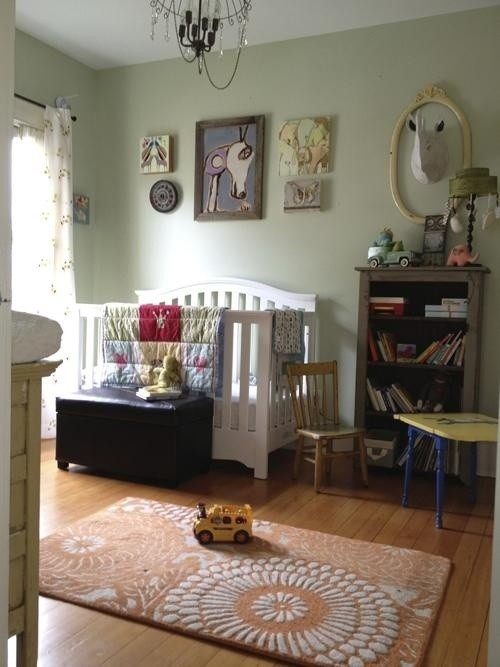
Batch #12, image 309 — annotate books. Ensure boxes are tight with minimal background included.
[135,390,180,400]
[138,386,181,396]
[369,291,467,478]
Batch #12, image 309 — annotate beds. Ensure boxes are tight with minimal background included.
[72,276,318,479]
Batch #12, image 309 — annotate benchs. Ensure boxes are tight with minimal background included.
[57,388,215,490]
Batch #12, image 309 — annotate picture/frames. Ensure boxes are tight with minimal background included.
[195,114,264,221]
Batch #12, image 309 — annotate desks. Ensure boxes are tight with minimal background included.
[393,409,499,528]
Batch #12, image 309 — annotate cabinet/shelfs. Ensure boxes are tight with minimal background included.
[353,263,481,478]
[10,359,55,667]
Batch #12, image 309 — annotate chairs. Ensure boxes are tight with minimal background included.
[286,358,371,494]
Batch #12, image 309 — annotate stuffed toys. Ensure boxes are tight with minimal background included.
[151,353,181,386]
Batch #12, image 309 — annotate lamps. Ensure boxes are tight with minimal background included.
[148,0,253,89]
[442,167,500,255]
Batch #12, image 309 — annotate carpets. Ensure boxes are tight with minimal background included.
[38,496,455,666]
